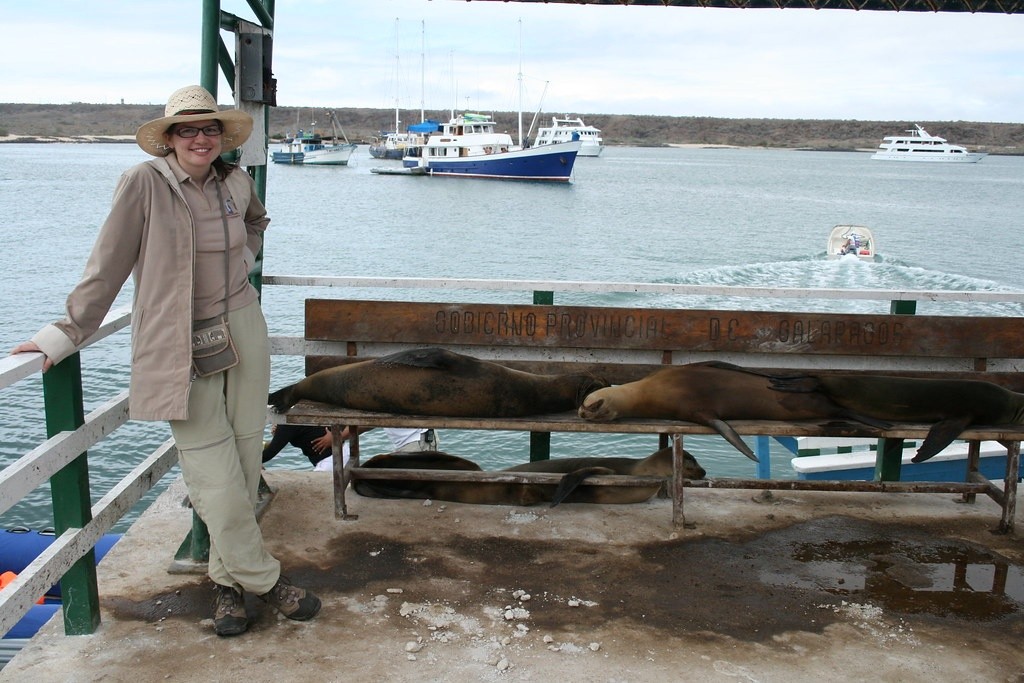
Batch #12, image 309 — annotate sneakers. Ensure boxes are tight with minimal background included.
[211,586,249,635]
[257,579,322,620]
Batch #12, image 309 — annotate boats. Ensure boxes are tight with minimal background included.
[371,166,430,174]
[871,124,989,164]
[827,224,875,262]
[407,85,583,183]
[792,435,1024,483]
[528,113,602,157]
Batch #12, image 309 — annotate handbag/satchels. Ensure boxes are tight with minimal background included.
[192,324,239,378]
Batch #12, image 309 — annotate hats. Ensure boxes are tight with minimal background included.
[136,84,253,156]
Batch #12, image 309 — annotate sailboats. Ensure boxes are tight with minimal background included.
[270,75,357,166]
[403,17,523,169]
[368,16,418,160]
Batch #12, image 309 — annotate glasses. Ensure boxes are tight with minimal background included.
[174,125,223,137]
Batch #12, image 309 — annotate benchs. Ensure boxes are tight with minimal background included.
[285,298,1024,533]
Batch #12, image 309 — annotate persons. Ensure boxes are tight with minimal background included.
[842,233,856,252]
[262,424,440,471]
[10,85,322,637]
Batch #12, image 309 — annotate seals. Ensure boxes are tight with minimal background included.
[266,347,1024,465]
[351,445,708,510]
